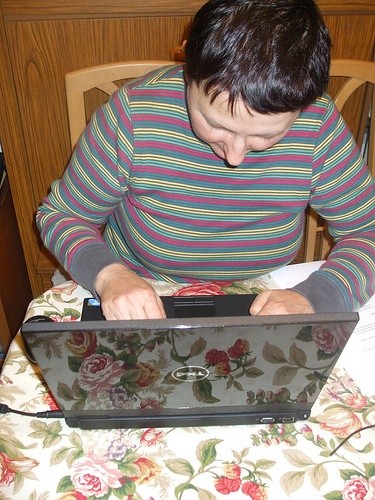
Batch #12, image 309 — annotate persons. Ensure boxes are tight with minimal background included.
[35,1,375,316]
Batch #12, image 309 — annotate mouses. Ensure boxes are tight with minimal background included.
[21,315,56,364]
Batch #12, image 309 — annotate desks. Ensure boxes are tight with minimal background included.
[0,260,374,498]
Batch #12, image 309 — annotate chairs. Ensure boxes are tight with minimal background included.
[64,59,186,154]
[301,58,374,263]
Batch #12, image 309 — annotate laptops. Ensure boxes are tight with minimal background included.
[19,293,361,429]
